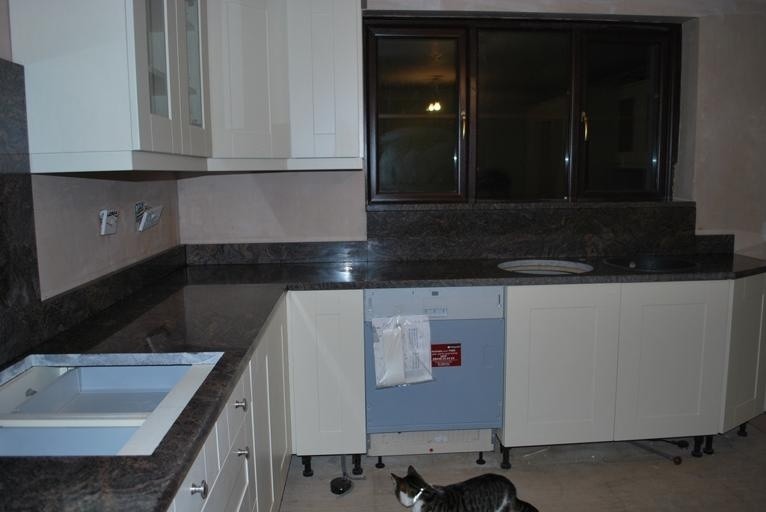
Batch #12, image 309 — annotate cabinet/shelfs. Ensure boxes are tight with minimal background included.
[363,11,472,213]
[194,0,370,177]
[7,2,212,181]
[615,270,732,461]
[247,289,294,512]
[718,259,766,439]
[493,278,622,472]
[475,13,684,212]
[283,285,368,478]
[161,360,260,512]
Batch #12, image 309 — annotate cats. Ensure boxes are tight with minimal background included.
[390,464,540,512]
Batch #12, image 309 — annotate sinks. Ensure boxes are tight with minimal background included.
[0,351,225,417]
[0,417,179,456]
[496,258,594,276]
